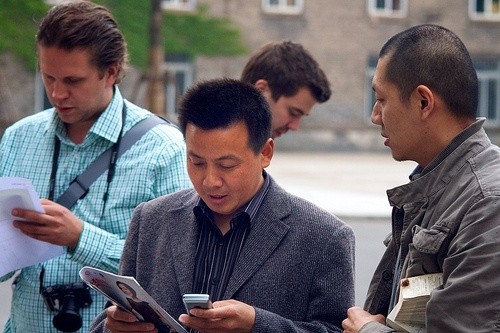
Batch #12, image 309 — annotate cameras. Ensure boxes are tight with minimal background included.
[40,282,94,333]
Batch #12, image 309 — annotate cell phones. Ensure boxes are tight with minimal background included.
[182,293,213,317]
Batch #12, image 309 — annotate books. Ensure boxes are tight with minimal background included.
[79,265,188,333]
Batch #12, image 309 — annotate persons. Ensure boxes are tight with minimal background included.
[115,280,177,333]
[0,0,194,333]
[240,40,332,138]
[85,78,356,333]
[340,24,500,333]
